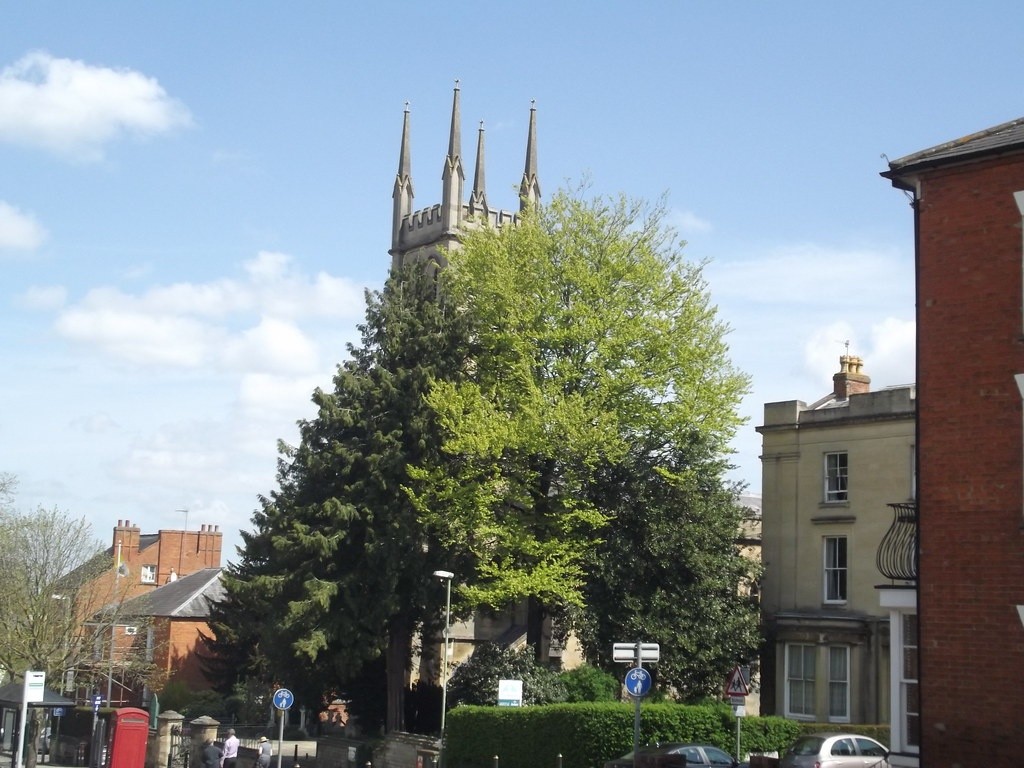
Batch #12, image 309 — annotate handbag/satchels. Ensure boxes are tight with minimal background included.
[252,755,264,768]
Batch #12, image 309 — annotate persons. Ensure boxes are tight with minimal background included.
[257,737,272,768]
[200,738,224,768]
[219,728,240,768]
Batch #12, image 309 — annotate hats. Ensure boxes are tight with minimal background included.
[258,736,269,743]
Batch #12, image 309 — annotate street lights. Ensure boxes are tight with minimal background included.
[52,594,71,741]
[433,569,457,746]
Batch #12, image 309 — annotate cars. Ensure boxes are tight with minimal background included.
[779,732,890,768]
[603,743,735,768]
